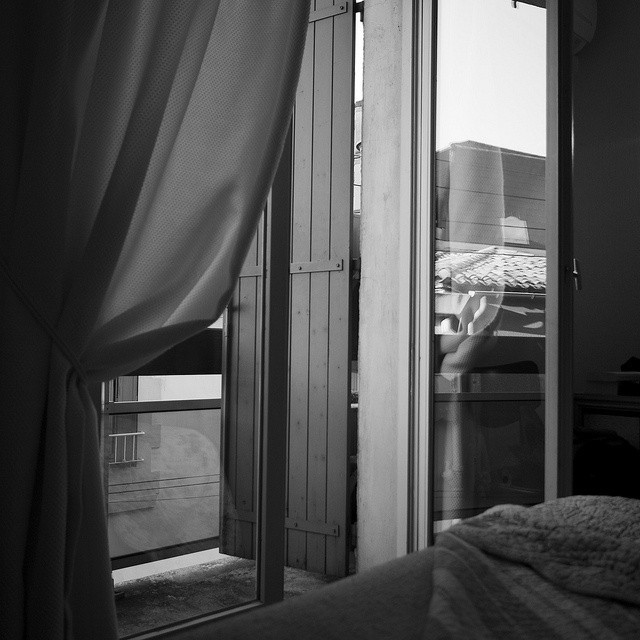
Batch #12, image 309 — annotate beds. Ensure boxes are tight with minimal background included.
[144,500,638,635]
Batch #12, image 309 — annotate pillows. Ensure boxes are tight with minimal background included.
[451,494,640,610]
